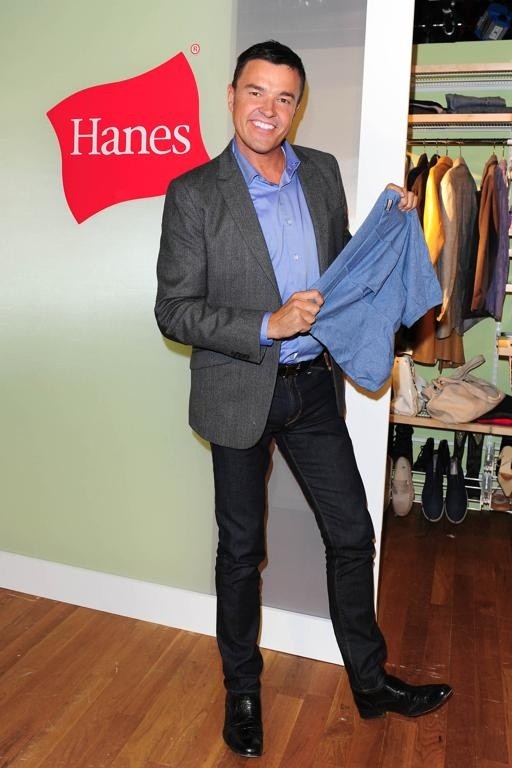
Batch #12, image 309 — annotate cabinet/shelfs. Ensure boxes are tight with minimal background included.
[390,64,511,505]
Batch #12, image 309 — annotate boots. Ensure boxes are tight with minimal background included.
[465,431,484,499]
[454,431,467,464]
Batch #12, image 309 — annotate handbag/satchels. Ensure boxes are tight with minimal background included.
[391,350,422,416]
[422,355,505,424]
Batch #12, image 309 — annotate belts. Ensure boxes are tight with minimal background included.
[276,356,327,379]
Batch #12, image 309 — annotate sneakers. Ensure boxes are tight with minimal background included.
[445,456,468,524]
[437,439,449,475]
[496,435,512,475]
[421,450,444,523]
[390,424,413,470]
[393,457,415,516]
[385,456,392,513]
[413,438,433,473]
[491,489,509,512]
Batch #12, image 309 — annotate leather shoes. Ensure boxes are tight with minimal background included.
[498,446,511,497]
[359,674,453,722]
[223,690,262,757]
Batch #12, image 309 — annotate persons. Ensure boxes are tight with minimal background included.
[154,39,453,757]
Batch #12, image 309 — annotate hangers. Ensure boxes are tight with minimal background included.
[406,139,510,161]
